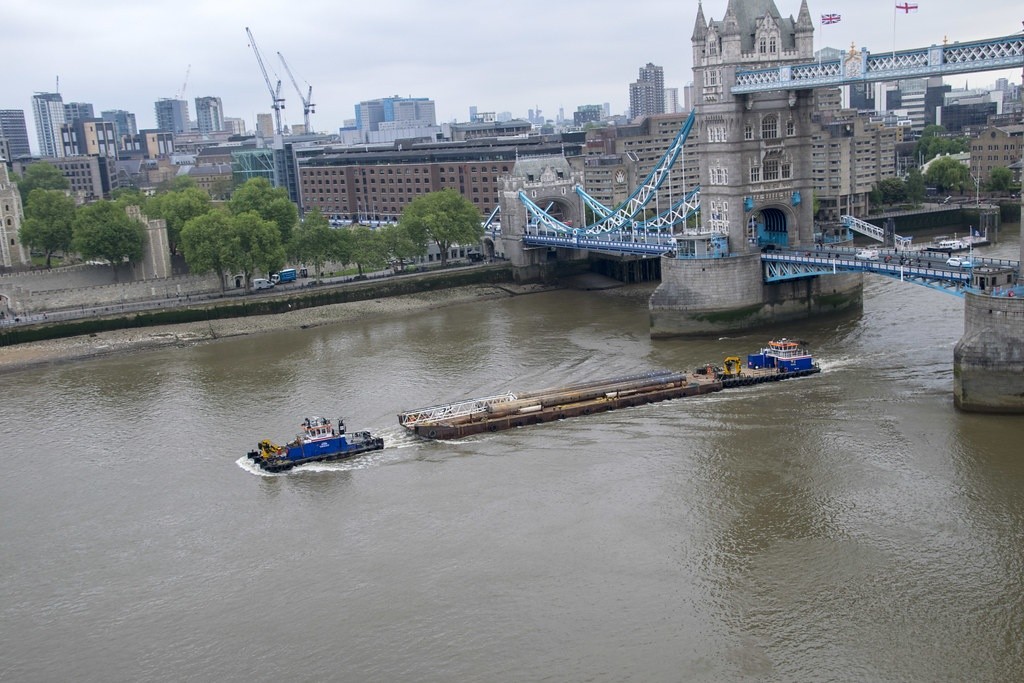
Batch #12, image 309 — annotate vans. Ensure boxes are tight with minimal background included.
[254,278,275,290]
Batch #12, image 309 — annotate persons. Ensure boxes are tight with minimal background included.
[828,251,830,258]
[884,256,892,264]
[948,250,952,258]
[927,260,932,268]
[894,247,897,254]
[816,253,819,257]
[899,256,921,267]
[805,250,811,257]
[835,253,840,259]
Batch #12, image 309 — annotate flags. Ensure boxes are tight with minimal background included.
[896,2,919,13]
[972,227,985,237]
[822,14,841,24]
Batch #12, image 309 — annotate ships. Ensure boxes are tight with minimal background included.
[397,335,822,442]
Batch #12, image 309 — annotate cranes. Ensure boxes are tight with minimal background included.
[276,51,317,135]
[244,26,287,137]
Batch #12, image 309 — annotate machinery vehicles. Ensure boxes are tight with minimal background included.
[716,356,743,381]
[257,439,287,463]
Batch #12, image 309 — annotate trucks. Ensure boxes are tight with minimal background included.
[271,269,297,285]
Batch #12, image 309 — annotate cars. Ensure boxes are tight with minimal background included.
[618,235,638,242]
[945,257,971,269]
[853,251,879,261]
[395,257,415,265]
[666,238,676,245]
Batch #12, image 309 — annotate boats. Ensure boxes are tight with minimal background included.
[246,414,385,476]
[962,225,992,247]
[927,231,972,252]
